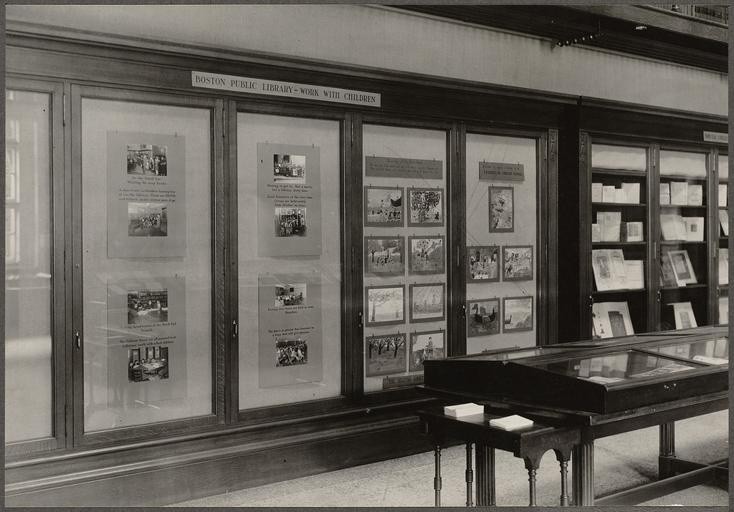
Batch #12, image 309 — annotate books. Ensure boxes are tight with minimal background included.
[588,178,727,341]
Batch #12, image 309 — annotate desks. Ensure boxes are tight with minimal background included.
[407,385,732,510]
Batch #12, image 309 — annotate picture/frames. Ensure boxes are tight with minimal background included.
[488,186,515,233]
[365,330,446,378]
[365,282,446,326]
[363,235,446,276]
[466,245,534,283]
[363,185,444,227]
[467,295,533,338]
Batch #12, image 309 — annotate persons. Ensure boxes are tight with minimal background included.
[125,142,169,238]
[272,153,306,239]
[274,282,308,368]
[124,288,169,384]
[410,334,443,369]
